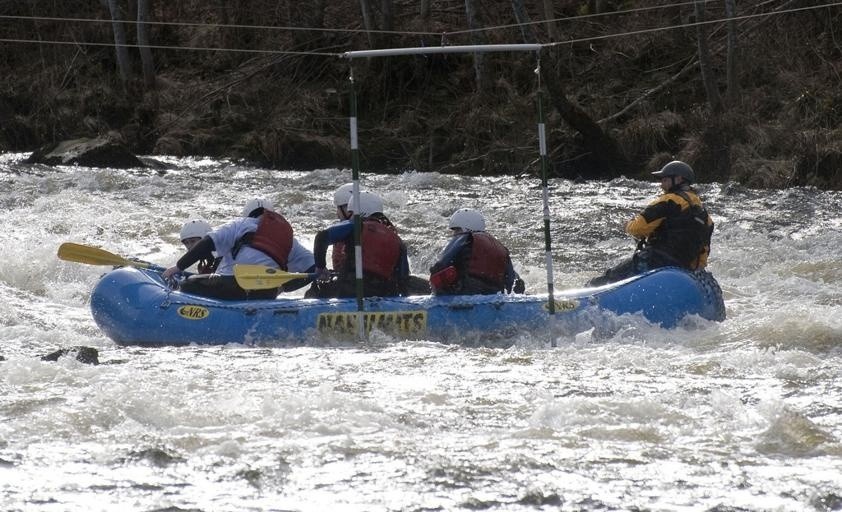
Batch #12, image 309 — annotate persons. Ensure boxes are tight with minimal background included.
[589,161,714,284]
[162,183,410,301]
[430,208,514,294]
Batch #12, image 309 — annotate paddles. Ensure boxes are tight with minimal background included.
[234,263,334,291]
[57,243,198,277]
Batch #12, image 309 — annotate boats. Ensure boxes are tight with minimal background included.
[90,262,726,348]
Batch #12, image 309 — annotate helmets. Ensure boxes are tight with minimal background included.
[180,219,213,242]
[243,198,273,217]
[334,182,364,206]
[651,161,695,183]
[449,208,485,233]
[347,191,383,217]
